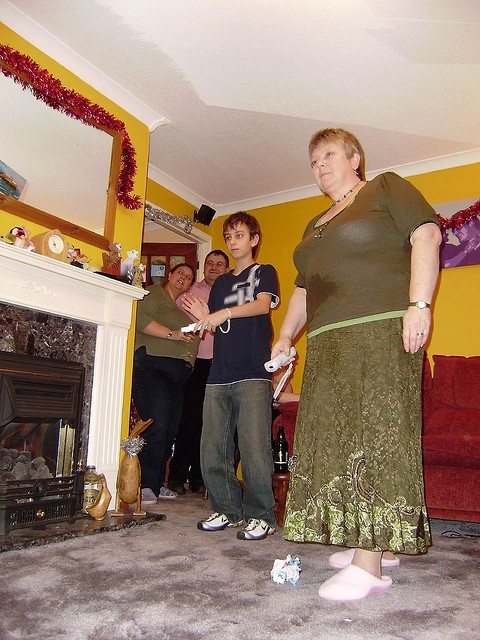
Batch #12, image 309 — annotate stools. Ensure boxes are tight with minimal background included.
[272,475,290,528]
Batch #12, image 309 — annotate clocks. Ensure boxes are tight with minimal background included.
[41,229,68,261]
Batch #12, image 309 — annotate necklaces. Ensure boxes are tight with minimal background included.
[331,179,361,206]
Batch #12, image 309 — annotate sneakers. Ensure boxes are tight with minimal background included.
[159,487,178,498]
[197,512,244,531]
[141,488,157,504]
[237,519,276,540]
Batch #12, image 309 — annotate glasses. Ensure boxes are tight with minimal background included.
[207,261,224,267]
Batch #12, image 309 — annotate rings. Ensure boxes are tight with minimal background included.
[417,333,424,336]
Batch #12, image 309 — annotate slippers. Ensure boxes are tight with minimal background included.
[329,549,399,568]
[318,564,392,600]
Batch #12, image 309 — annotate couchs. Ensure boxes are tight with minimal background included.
[273,349,480,522]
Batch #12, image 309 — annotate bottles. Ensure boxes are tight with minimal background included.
[82,463,99,514]
[273,425,288,472]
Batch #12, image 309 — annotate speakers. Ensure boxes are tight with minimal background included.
[196,203,216,226]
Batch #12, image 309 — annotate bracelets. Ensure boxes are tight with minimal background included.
[166,330,173,340]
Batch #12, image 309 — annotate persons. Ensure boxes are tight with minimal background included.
[132,263,200,504]
[181,211,280,540]
[175,250,230,495]
[270,128,443,603]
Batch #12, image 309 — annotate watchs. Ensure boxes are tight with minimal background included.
[408,300,432,311]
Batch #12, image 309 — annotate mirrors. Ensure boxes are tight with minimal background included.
[1,56,124,253]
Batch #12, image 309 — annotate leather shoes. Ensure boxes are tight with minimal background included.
[189,482,206,493]
[167,481,185,495]
